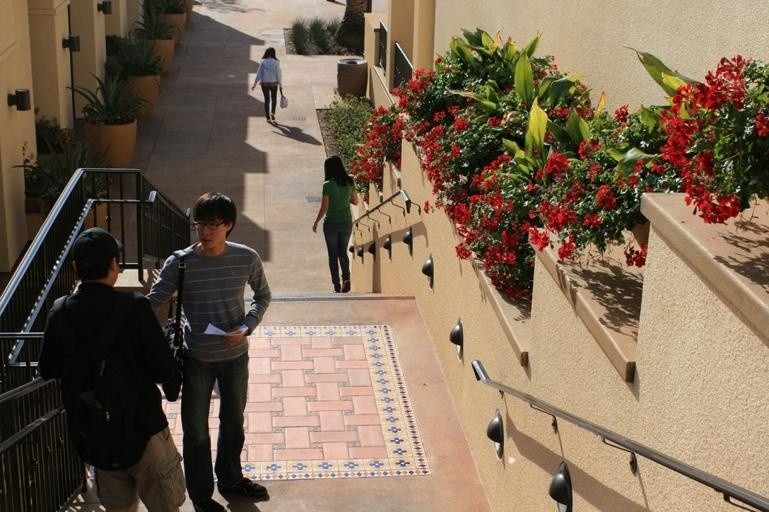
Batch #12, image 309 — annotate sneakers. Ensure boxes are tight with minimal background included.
[270,113,275,120]
[191,497,224,511]
[342,278,351,293]
[266,118,270,123]
[217,477,266,500]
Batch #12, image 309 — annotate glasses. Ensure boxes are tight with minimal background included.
[191,221,222,230]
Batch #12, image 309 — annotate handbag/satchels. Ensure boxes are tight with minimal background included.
[280,97,287,108]
[159,250,186,401]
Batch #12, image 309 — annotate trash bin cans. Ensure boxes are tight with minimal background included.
[337,58,368,101]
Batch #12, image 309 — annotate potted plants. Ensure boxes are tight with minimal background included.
[106,33,166,120]
[66,63,154,168]
[135,2,187,67]
[23,106,111,231]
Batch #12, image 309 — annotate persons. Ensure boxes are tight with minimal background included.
[312,155,358,292]
[38,227,185,512]
[147,192,271,512]
[252,47,284,120]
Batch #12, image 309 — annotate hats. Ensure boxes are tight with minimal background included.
[72,227,124,269]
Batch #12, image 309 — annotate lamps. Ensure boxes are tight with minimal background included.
[9,88,31,111]
